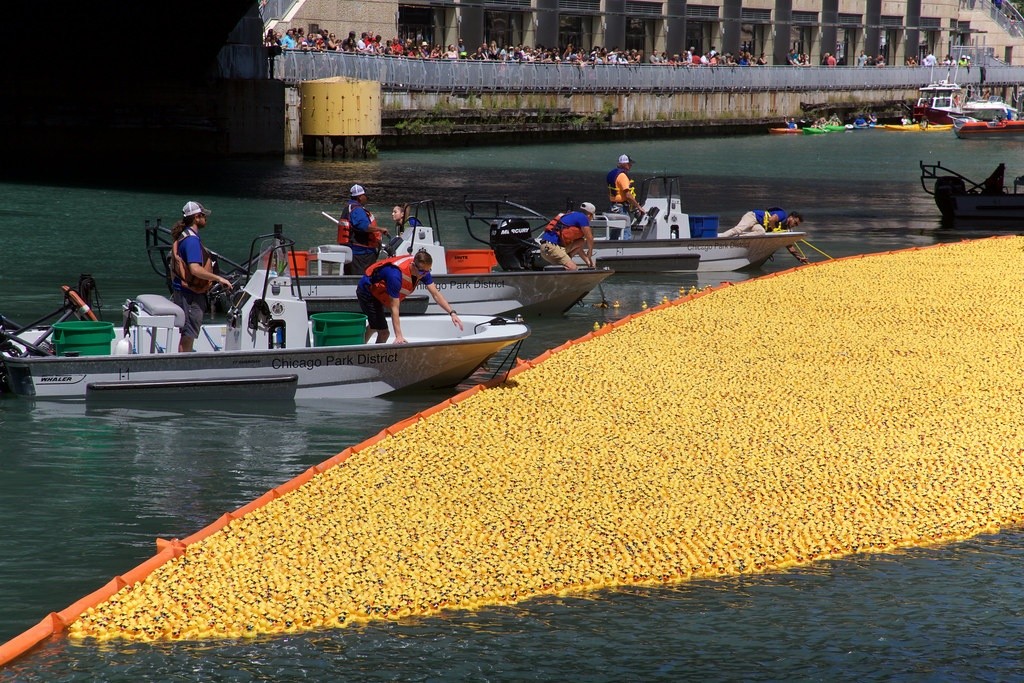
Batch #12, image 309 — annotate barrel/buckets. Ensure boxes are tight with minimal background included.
[310,312,369,348]
[689,215,719,238]
[264,251,308,276]
[446,249,498,274]
[51,321,117,356]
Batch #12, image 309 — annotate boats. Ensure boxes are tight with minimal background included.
[768,127,802,133]
[905,42,1018,123]
[848,123,953,132]
[827,124,847,132]
[464,173,806,272]
[803,128,829,133]
[0,224,529,400]
[291,197,614,320]
[952,115,1024,138]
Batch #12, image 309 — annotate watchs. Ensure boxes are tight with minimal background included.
[450,310,457,315]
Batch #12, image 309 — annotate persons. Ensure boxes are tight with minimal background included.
[607,154,646,241]
[717,206,809,264]
[901,115,929,131]
[356,250,464,346]
[784,111,877,130]
[171,201,234,352]
[337,183,425,275]
[967,89,991,102]
[991,0,1024,35]
[785,48,887,67]
[992,107,1011,126]
[904,50,972,68]
[920,100,932,108]
[263,28,768,68]
[540,202,596,272]
[962,0,975,10]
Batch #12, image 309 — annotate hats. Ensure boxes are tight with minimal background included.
[617,153,636,164]
[421,42,429,46]
[580,202,596,220]
[182,201,211,217]
[350,184,369,196]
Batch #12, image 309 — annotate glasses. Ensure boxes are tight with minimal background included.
[792,219,799,227]
[415,264,432,274]
